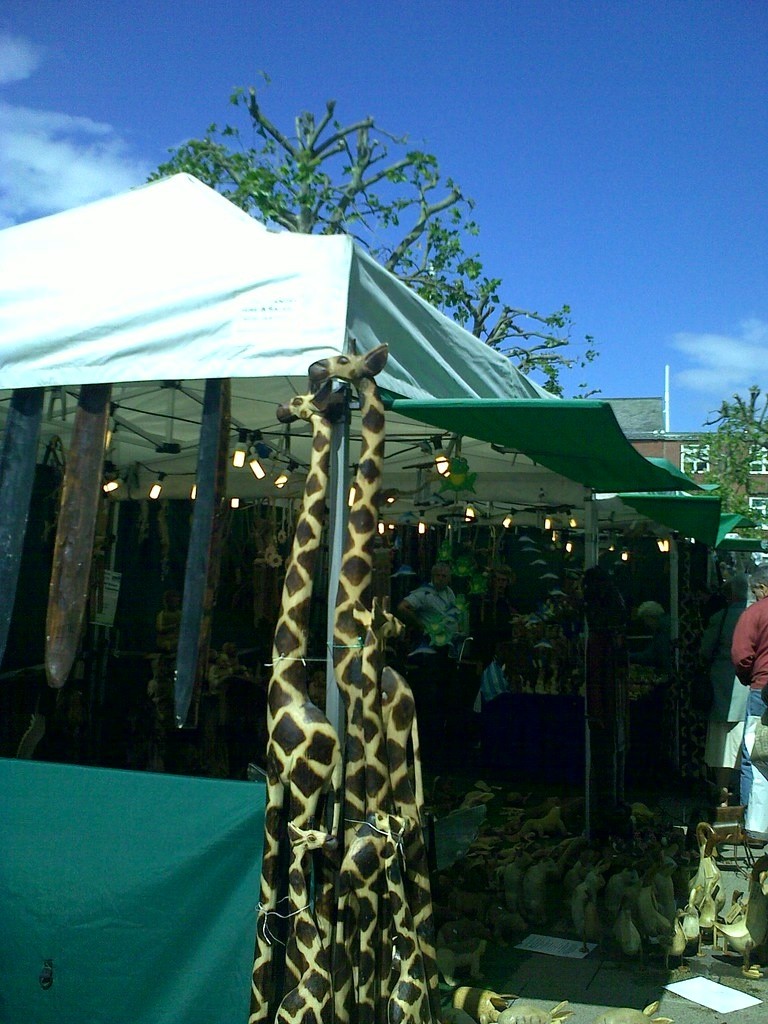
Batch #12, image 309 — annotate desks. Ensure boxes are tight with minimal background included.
[475,675,675,788]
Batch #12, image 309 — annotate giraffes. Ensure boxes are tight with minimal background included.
[249,342,506,1024]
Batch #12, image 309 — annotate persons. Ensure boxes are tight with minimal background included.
[398,562,768,850]
[157,589,182,632]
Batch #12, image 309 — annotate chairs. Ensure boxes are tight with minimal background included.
[713,806,768,879]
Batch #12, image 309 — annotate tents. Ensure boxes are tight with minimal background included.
[0,174,768,560]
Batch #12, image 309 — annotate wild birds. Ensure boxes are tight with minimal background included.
[431,773,768,1024]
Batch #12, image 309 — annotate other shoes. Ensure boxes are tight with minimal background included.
[742,831,764,849]
